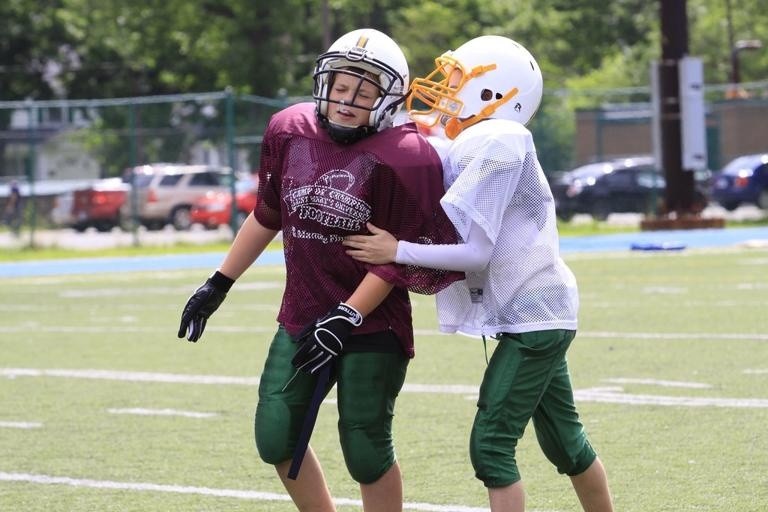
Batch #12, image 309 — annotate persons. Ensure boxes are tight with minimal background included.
[174,26,467,512]
[338,29,614,511]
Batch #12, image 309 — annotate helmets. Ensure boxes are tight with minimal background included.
[312,29,409,140]
[408,36,544,141]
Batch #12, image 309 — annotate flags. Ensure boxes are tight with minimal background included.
[1,183,21,241]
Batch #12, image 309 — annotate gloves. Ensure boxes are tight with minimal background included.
[175,270,236,343]
[291,301,364,377]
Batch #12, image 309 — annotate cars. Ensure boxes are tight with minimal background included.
[543,149,768,222]
[46,151,265,234]
